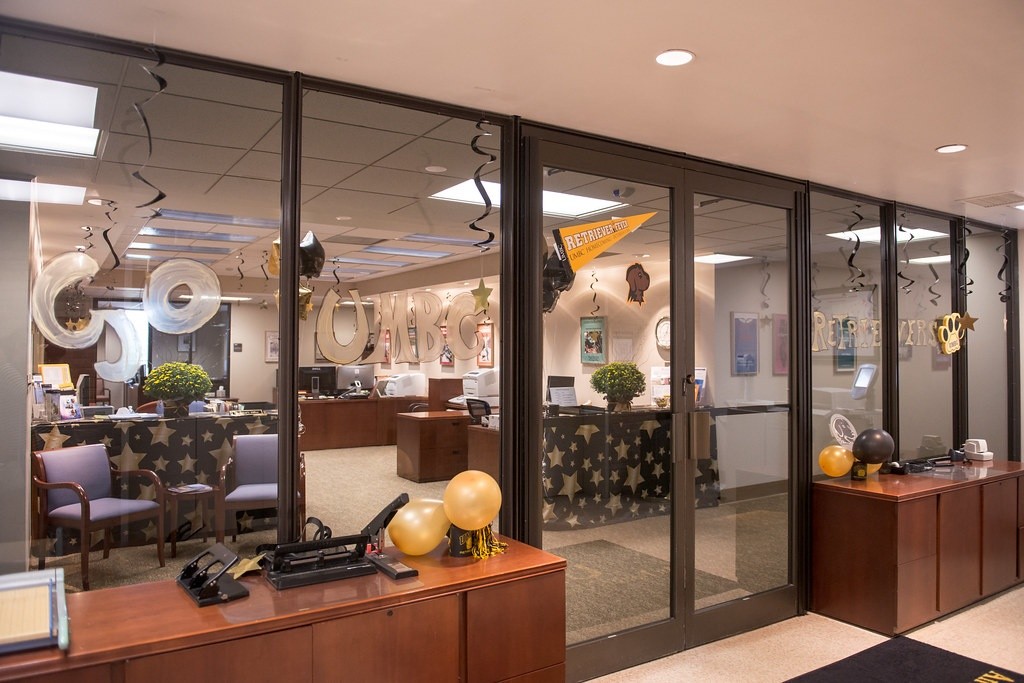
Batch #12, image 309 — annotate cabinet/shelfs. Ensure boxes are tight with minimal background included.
[396,408,500,482]
[816,459,1024,651]
[1,534,568,683]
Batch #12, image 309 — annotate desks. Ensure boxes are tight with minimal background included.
[299,397,430,450]
[163,481,219,557]
[470,401,793,530]
[29,405,300,558]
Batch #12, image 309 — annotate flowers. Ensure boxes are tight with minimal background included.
[141,363,212,398]
[591,361,647,398]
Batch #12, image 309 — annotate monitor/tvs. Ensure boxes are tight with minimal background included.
[546,376,574,401]
[298,364,375,400]
[851,364,879,411]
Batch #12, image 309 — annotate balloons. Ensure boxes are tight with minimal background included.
[852,427,894,473]
[387,497,452,555]
[443,470,502,531]
[818,445,855,477]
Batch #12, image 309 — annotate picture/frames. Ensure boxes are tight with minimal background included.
[176,281,952,380]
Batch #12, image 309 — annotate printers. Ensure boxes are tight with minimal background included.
[382,372,430,397]
[446,367,499,409]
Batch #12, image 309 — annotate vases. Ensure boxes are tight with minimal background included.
[164,397,189,419]
[608,394,636,413]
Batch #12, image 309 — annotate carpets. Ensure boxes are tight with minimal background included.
[779,632,1024,683]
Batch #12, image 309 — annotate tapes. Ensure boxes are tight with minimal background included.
[955,451,965,454]
[891,462,900,467]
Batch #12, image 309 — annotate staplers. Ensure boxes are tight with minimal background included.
[358,493,420,580]
[928,456,954,467]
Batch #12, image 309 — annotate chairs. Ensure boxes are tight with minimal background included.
[97,377,111,405]
[31,443,165,590]
[216,432,288,547]
